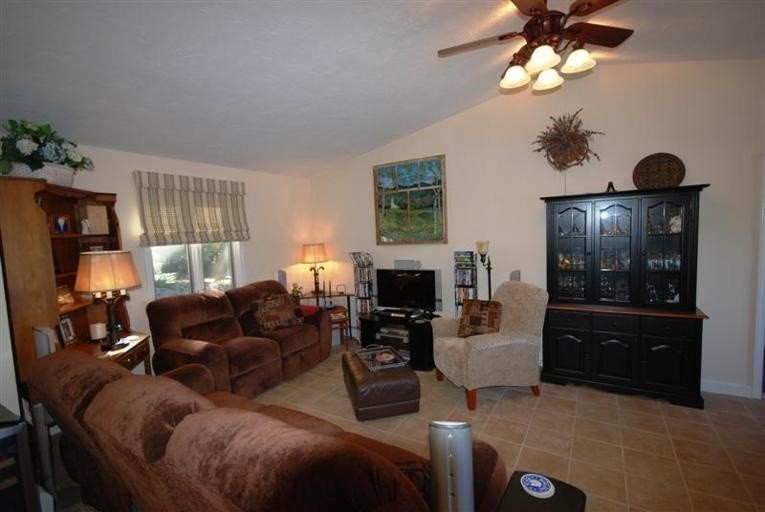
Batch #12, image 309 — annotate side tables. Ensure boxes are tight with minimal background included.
[302,292,355,340]
[496,470,586,512]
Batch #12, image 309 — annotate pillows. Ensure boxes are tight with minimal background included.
[458,298,502,337]
[251,293,304,333]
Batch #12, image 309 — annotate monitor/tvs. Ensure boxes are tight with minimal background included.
[376,268,436,315]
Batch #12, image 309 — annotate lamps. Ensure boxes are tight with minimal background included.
[75,251,142,350]
[500,11,596,90]
[475,241,492,300]
[302,243,328,293]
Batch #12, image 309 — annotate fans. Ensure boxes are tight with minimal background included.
[438,0,634,80]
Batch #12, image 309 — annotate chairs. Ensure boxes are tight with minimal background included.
[0,419,38,511]
[431,281,549,410]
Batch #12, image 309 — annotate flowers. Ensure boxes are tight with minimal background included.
[0,119,95,174]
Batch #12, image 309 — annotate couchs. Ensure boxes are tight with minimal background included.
[146,280,331,399]
[28,348,506,511]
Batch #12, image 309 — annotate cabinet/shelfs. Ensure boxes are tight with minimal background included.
[0,177,153,477]
[359,313,441,371]
[454,250,477,306]
[538,183,711,410]
[349,252,373,313]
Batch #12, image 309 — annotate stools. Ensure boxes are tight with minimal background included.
[331,313,349,350]
[342,352,419,421]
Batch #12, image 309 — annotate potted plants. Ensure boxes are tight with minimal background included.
[292,283,303,304]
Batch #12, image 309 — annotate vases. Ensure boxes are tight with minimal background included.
[10,161,74,188]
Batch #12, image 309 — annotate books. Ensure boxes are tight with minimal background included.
[349,252,375,313]
[453,250,477,317]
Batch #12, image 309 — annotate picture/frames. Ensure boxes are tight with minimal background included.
[373,154,448,246]
[59,315,77,346]
